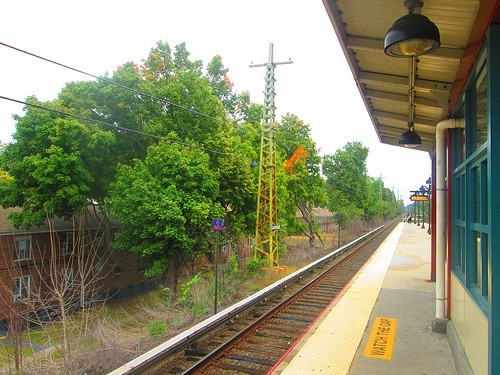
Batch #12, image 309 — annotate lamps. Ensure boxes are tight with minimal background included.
[382,0,442,60]
[398,120,422,147]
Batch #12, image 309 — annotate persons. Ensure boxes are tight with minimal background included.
[402,210,413,224]
